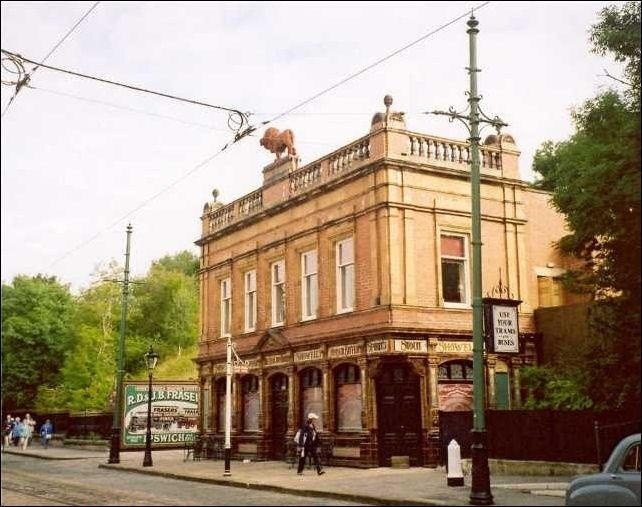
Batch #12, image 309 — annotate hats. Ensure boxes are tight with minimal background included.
[307,413,319,420]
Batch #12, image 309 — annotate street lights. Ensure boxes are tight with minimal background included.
[143,345,158,467]
[216,332,249,476]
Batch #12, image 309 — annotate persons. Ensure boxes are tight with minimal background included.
[294,411,326,477]
[40,418,54,448]
[3,412,36,451]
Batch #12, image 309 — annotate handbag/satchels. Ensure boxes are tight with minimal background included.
[293,431,308,446]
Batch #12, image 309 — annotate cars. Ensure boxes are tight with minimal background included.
[564,432,641,505]
[127,405,200,432]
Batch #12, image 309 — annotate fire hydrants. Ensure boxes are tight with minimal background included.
[444,438,465,486]
